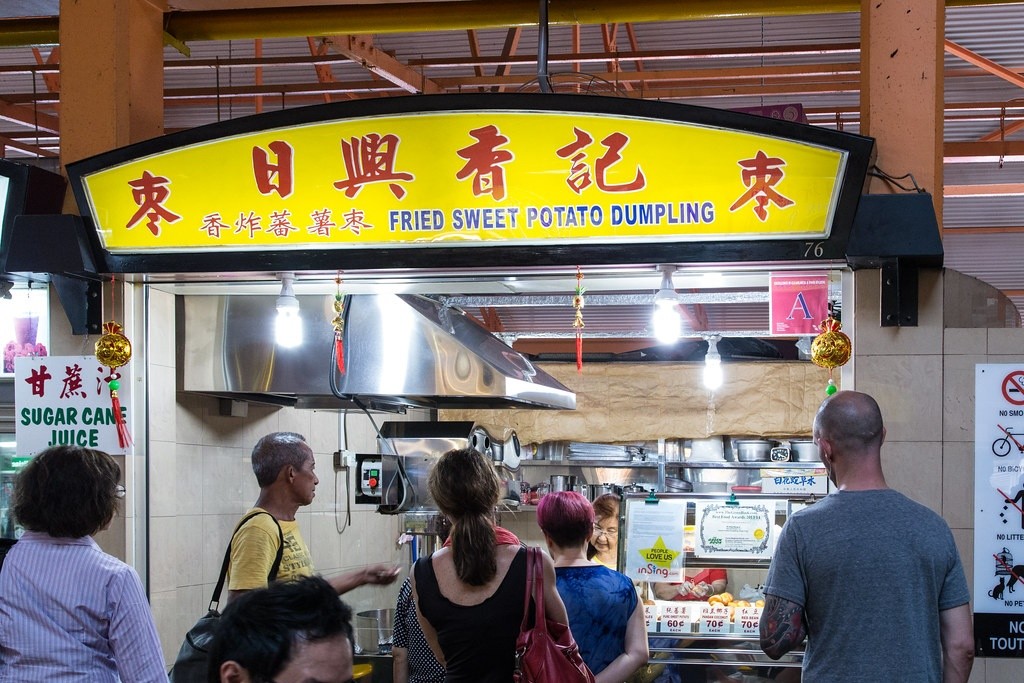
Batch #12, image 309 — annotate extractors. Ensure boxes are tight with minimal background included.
[224,294,578,413]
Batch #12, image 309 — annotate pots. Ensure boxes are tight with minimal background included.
[356,608,396,653]
[550,474,644,504]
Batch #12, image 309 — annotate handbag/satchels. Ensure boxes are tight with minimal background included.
[168,512,283,683]
[514,547,596,683]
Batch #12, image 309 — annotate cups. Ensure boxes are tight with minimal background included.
[497,481,550,506]
[548,441,564,460]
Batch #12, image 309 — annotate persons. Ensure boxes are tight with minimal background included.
[537,490,648,683]
[226,432,400,596]
[654,568,729,600]
[759,391,974,683]
[211,576,355,683]
[408,448,571,683]
[586,493,645,600]
[391,515,453,683]
[0,446,166,683]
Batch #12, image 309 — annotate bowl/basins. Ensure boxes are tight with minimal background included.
[788,440,822,462]
[734,439,782,462]
[664,477,692,493]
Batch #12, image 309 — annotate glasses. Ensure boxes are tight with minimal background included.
[592,528,618,540]
[115,484,126,498]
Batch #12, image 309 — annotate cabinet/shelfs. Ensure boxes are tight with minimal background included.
[518,439,829,512]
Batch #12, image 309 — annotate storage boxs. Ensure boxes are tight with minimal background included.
[760,469,828,494]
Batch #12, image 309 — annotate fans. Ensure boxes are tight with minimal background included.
[516,0,627,97]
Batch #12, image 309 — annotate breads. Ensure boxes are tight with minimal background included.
[707,593,765,623]
